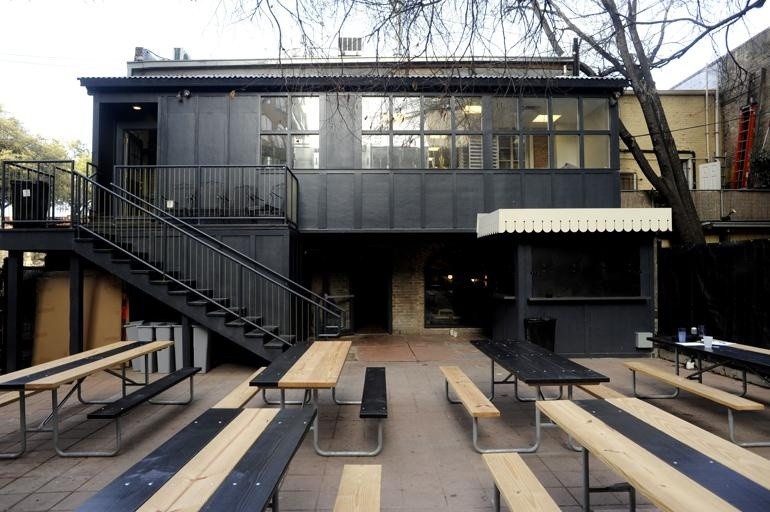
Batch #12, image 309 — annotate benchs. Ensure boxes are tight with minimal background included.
[0,340,203,461]
[211,340,388,457]
[481,453,563,512]
[439,365,501,453]
[620,360,766,449]
[333,464,383,511]
[576,382,629,399]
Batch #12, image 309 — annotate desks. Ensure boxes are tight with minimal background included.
[73,406,319,512]
[470,337,611,452]
[535,397,770,512]
[645,330,770,448]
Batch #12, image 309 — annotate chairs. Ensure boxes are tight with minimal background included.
[160,181,286,217]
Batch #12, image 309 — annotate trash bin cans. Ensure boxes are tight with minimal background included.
[124,320,209,374]
[10,179,49,229]
[524,317,558,352]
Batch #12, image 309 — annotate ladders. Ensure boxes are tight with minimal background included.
[732,67,764,189]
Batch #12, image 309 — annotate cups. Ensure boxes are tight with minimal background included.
[703,336,713,348]
[678,328,687,342]
